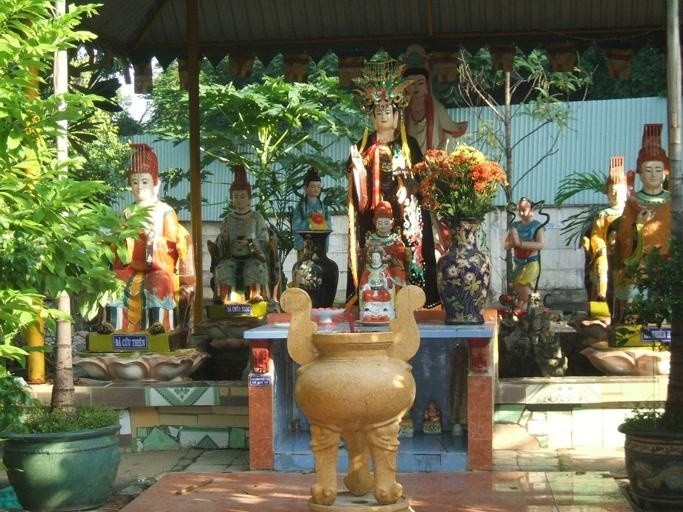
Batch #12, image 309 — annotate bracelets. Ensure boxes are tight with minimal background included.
[518,239,526,251]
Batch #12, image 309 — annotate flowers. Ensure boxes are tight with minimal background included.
[414,140,508,218]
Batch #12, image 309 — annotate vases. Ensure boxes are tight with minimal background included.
[290,230,339,306]
[436,222,491,325]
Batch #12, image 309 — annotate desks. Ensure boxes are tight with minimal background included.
[242,309,501,474]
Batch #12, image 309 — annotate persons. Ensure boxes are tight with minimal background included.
[292,167,331,262]
[365,201,404,277]
[99,163,191,334]
[399,64,467,259]
[348,95,438,308]
[582,174,627,301]
[424,399,441,422]
[614,147,670,322]
[505,198,545,307]
[360,240,392,291]
[207,182,269,306]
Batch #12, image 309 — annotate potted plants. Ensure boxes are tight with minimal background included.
[616,232,683,512]
[0,404,123,511]
